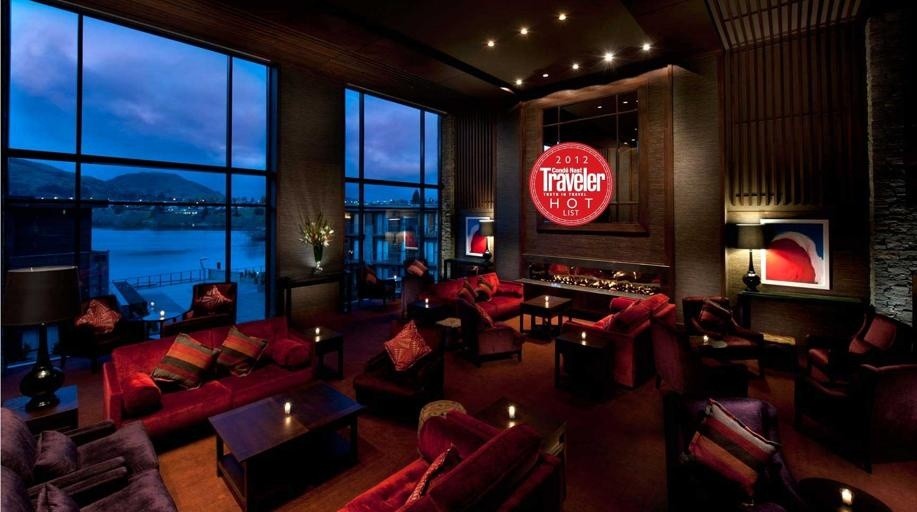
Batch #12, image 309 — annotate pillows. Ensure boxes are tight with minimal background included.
[458,277,494,304]
[151,326,268,393]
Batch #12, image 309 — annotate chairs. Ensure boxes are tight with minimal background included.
[563,293,676,389]
[1,403,177,512]
[74,281,236,374]
[360,259,434,312]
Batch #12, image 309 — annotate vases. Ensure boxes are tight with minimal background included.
[312,244,323,274]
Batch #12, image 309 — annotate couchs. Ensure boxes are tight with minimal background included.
[420,272,525,321]
[102,314,320,440]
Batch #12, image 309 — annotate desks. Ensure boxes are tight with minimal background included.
[555,328,616,406]
[281,268,353,324]
[208,382,366,512]
[444,256,486,281]
[473,398,566,498]
[518,295,571,338]
[1,383,79,441]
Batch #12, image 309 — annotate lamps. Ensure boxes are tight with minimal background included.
[731,223,769,294]
[1,265,79,409]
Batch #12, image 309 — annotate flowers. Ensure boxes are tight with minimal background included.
[297,210,335,247]
